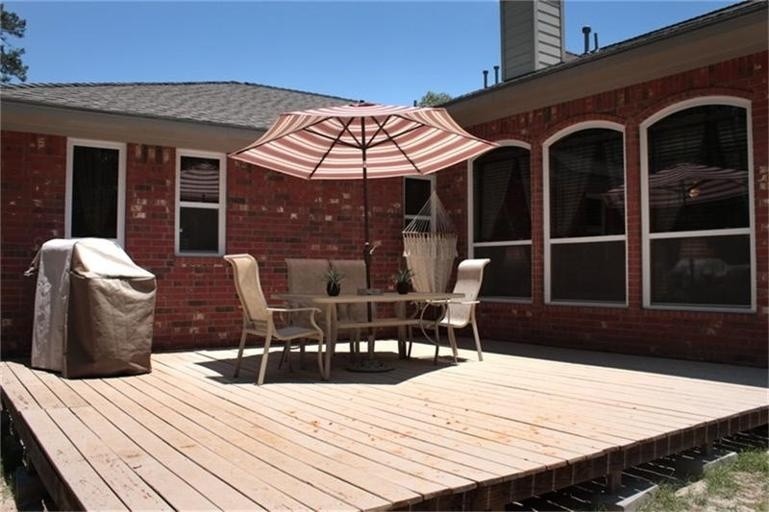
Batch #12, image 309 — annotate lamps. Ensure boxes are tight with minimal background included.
[222,252,492,385]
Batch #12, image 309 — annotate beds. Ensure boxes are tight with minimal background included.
[180,162,219,201]
[602,165,749,281]
[227,98,501,360]
